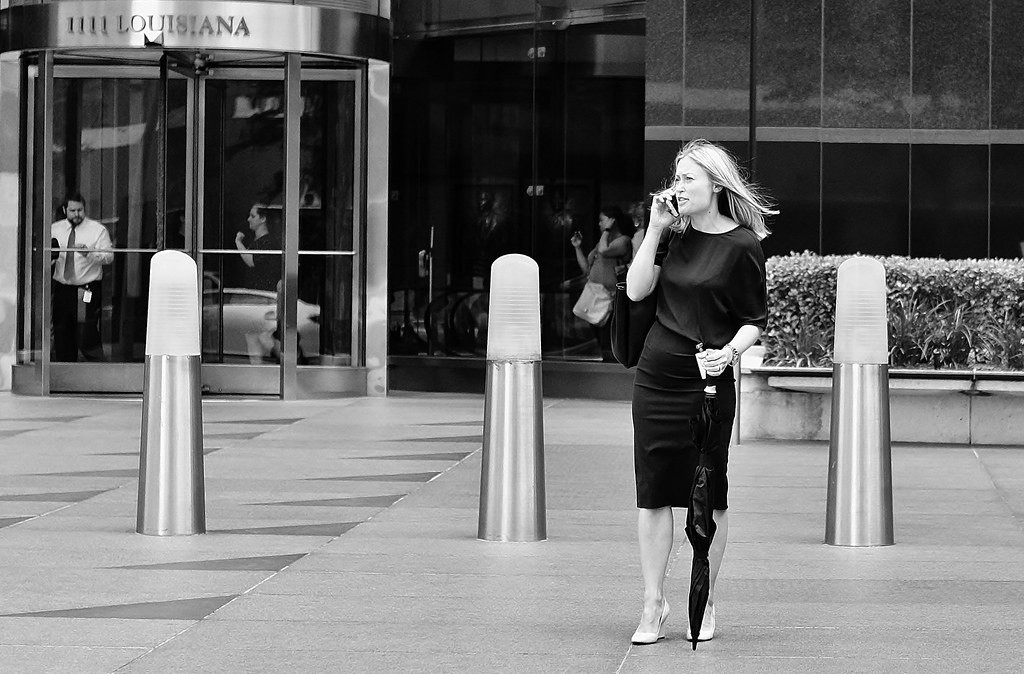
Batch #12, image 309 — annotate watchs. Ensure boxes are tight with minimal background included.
[723,344,739,366]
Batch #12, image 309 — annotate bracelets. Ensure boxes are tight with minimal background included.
[603,228,611,232]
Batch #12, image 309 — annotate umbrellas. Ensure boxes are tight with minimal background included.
[685,376,722,650]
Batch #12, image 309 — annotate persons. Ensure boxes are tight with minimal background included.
[482,192,513,294]
[631,141,772,645]
[51,193,115,361]
[570,206,631,363]
[235,203,280,365]
[630,199,643,258]
[174,211,185,247]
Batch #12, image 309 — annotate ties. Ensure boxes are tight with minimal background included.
[64,223,77,281]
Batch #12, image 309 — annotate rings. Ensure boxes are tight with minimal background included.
[718,366,721,371]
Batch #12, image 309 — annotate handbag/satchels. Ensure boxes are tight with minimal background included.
[610,229,676,370]
[51,238,59,261]
[573,278,616,328]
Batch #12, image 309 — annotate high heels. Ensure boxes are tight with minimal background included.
[631,596,671,644]
[687,601,715,641]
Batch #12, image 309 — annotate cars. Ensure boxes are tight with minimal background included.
[401,312,478,344]
[87,270,320,363]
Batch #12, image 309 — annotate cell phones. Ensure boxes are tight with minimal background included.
[666,195,680,218]
[576,232,582,240]
